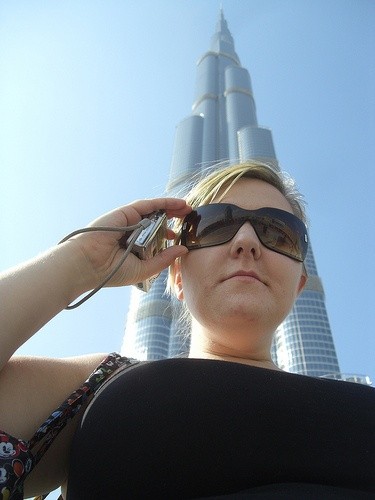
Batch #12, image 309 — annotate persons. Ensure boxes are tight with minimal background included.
[0,159,375,500]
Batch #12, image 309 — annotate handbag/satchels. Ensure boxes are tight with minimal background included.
[0,352,128,500]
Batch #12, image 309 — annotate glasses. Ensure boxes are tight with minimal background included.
[180,203,308,262]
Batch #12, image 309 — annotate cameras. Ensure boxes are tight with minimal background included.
[126,210,167,292]
